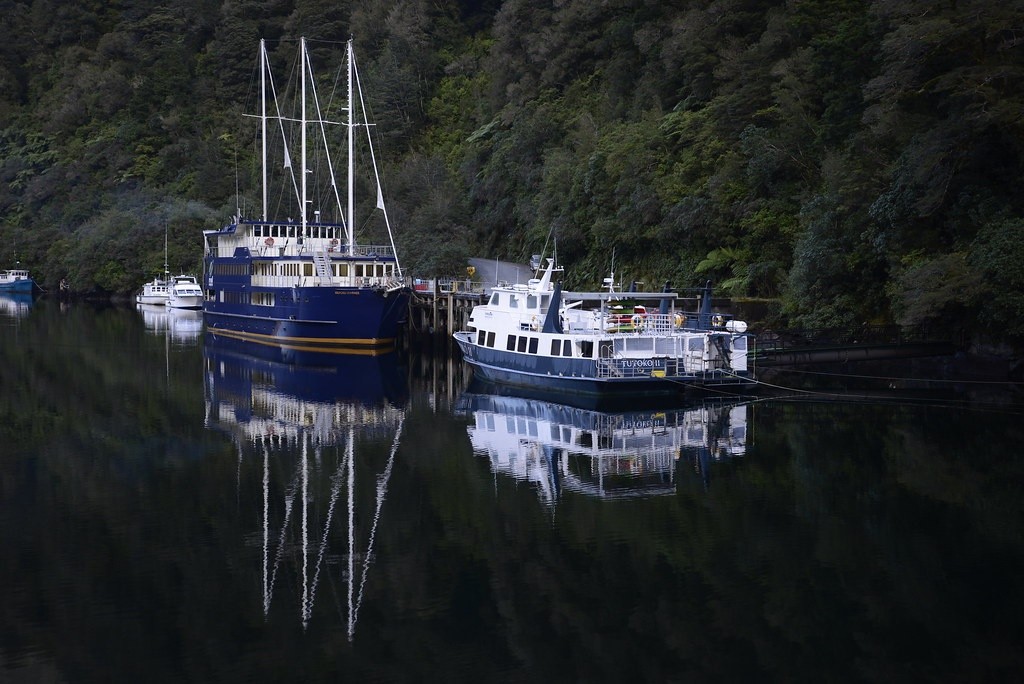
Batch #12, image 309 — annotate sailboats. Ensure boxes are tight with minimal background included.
[200,31,416,355]
[201,351,418,641]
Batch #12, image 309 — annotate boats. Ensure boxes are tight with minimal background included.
[450,369,755,532]
[0,237,34,291]
[448,222,758,398]
[134,221,173,305]
[170,266,204,311]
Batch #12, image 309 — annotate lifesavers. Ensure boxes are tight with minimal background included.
[674,314,681,329]
[331,239,338,247]
[265,238,274,247]
[712,313,723,327]
[631,315,644,330]
[531,315,539,330]
